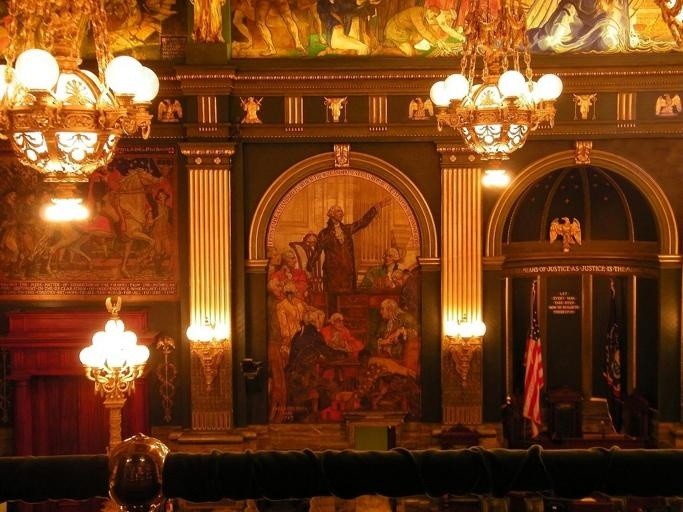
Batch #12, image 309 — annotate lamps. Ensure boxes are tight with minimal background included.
[427,0,566,196]
[75,292,156,512]
[0,0,166,225]
[442,312,488,394]
[184,315,235,397]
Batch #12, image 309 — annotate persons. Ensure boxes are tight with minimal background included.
[267,198,421,423]
[0,183,174,275]
[231,0,467,59]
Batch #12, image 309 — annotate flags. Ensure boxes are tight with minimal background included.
[602,283,622,406]
[521,282,546,439]
